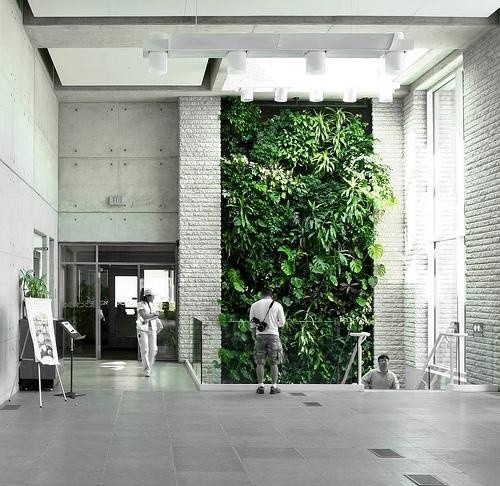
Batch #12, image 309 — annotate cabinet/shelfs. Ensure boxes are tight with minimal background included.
[16,318,63,393]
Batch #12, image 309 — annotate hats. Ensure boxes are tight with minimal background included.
[143,288,156,296]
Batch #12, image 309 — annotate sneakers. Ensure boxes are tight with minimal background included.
[256,386,282,395]
[144,369,152,377]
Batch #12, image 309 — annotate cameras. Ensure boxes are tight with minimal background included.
[250,317,267,332]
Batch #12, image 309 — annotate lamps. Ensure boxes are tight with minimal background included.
[141,0,416,77]
[232,79,404,106]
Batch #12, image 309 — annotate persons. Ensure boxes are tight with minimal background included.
[361,354,400,389]
[135,288,163,377]
[249,288,286,395]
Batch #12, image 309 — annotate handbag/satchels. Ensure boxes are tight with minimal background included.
[156,319,163,334]
